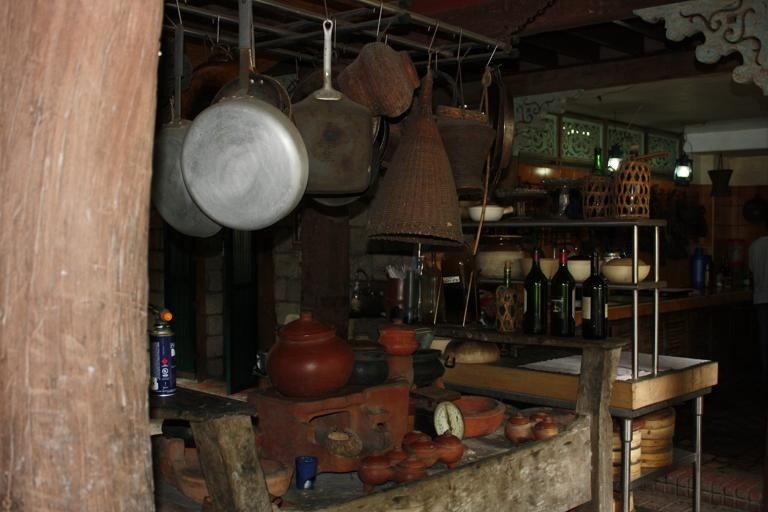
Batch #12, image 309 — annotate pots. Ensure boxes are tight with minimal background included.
[444,339,502,368]
[211,1,294,122]
[151,3,223,237]
[288,19,377,217]
[183,0,310,235]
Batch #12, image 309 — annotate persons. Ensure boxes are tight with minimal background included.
[747,211,767,401]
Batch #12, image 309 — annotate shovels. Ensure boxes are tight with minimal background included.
[287,18,373,194]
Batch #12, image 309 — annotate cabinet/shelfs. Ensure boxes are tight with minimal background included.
[462,220,719,512]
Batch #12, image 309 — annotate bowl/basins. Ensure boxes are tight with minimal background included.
[452,395,506,438]
[176,454,294,503]
[474,244,605,282]
[468,206,503,222]
[602,265,652,286]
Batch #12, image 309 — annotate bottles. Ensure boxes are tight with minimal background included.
[150,316,177,398]
[356,411,558,488]
[266,310,445,397]
[402,245,445,325]
[497,248,609,340]
[691,247,733,289]
[584,144,652,220]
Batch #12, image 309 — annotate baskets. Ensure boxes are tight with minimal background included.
[495,285,522,333]
[613,151,671,222]
[581,174,613,221]
[434,103,487,122]
[336,41,420,119]
[367,72,466,248]
[439,122,497,191]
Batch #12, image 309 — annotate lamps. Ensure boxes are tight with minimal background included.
[673,133,693,186]
[607,112,623,172]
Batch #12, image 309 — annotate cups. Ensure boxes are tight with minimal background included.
[296,454,318,492]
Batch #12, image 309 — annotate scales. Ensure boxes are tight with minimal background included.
[408,383,467,445]
